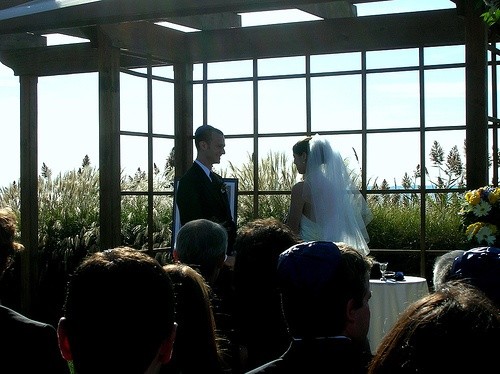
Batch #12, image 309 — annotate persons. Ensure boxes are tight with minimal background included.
[287,133,375,257]
[0,208,500,374]
[177,125,237,257]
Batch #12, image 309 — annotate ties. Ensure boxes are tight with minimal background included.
[209,171,221,185]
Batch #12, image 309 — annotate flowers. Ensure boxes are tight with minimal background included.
[458,185,500,247]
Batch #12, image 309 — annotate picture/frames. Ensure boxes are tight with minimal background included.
[171,178,238,253]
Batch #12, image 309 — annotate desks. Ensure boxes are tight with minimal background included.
[368,275,428,355]
